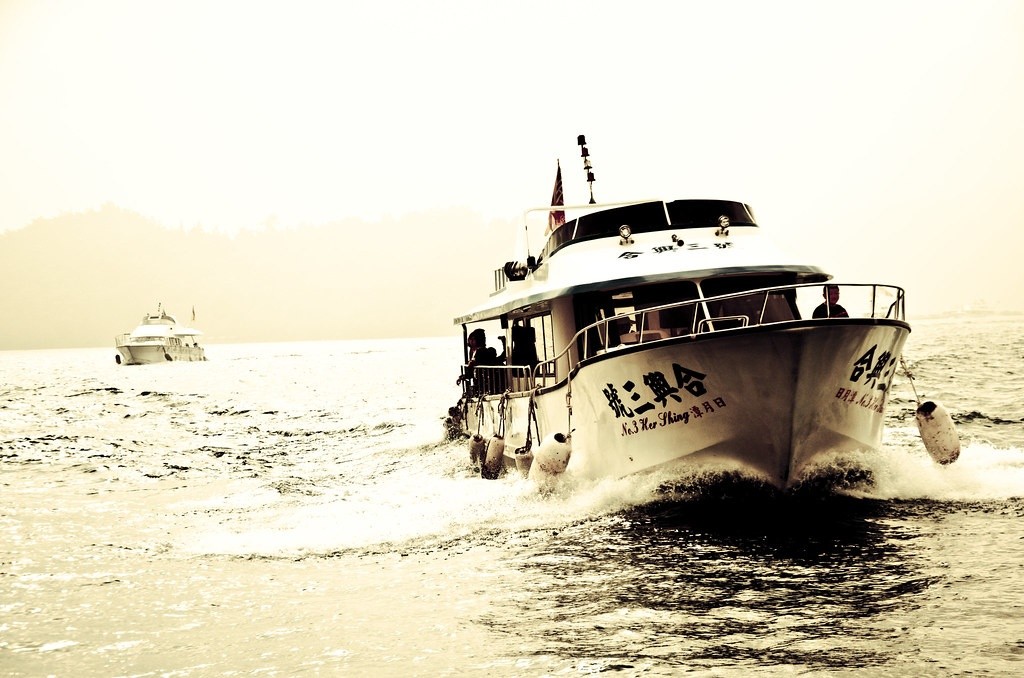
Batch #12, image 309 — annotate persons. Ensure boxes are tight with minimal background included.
[617,313,636,335]
[456,328,508,395]
[812,286,849,318]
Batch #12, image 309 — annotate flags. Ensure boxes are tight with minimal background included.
[551,168,566,225]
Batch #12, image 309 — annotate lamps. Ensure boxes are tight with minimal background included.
[584,160,592,169]
[619,225,631,243]
[719,215,729,234]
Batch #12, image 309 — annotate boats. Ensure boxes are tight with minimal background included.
[449,135,912,501]
[115,302,208,365]
